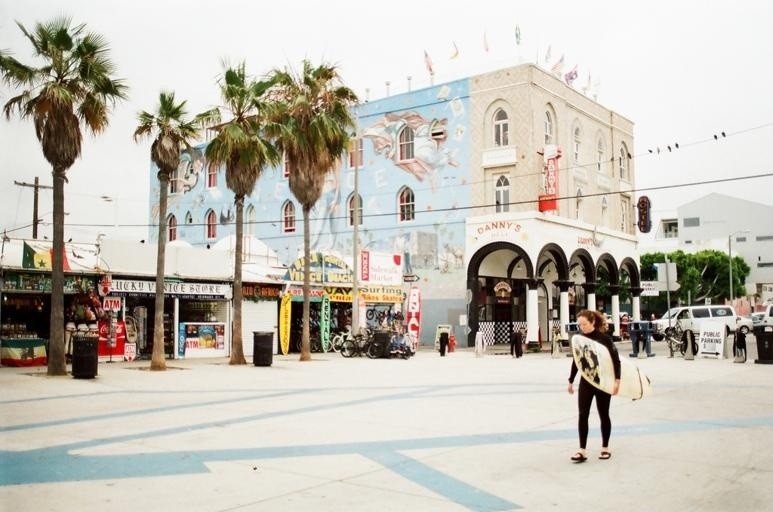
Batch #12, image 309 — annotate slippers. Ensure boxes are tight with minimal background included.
[598,452,612,460]
[571,452,588,461]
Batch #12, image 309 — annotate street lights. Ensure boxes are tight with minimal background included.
[349,110,378,338]
[728,230,752,301]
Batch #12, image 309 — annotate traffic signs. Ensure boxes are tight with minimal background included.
[403,275,419,283]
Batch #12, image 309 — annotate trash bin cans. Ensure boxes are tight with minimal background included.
[72,336,98,379]
[253,332,273,366]
[754,325,773,363]
[630,321,655,357]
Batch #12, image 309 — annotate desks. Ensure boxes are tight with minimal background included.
[0,338,48,368]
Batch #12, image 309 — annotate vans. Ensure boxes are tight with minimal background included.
[651,304,737,340]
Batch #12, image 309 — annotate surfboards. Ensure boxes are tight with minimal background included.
[571,334,652,400]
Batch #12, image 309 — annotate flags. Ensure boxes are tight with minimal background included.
[423,24,579,85]
[22,241,53,269]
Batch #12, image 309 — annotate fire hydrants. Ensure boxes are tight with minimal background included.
[448,335,456,353]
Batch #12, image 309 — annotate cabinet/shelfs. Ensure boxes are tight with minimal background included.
[140,311,175,359]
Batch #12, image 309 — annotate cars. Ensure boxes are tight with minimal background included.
[733,304,773,334]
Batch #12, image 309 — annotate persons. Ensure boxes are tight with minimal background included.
[567,310,622,462]
[651,313,657,321]
[622,314,628,322]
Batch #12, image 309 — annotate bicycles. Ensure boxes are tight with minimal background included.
[666,319,698,355]
[291,303,415,361]
